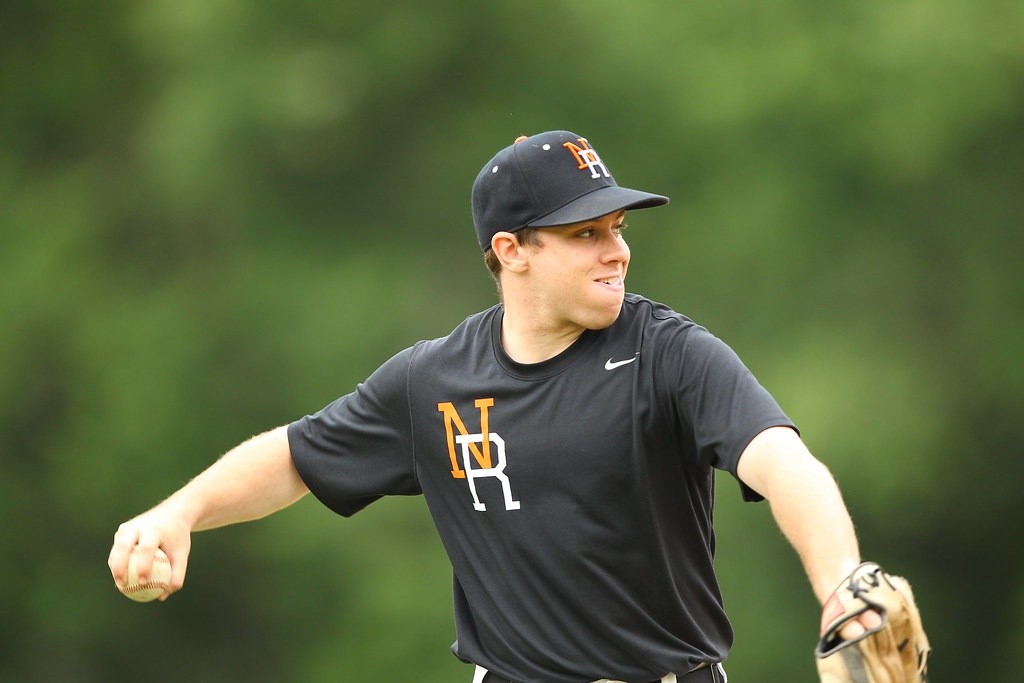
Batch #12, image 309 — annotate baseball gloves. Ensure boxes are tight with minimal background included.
[811,561,933,683]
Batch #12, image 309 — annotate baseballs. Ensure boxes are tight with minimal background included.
[112,546,171,602]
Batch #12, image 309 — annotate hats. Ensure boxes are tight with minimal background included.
[470,130,669,253]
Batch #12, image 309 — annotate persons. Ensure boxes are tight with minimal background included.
[108,131,931,682]
